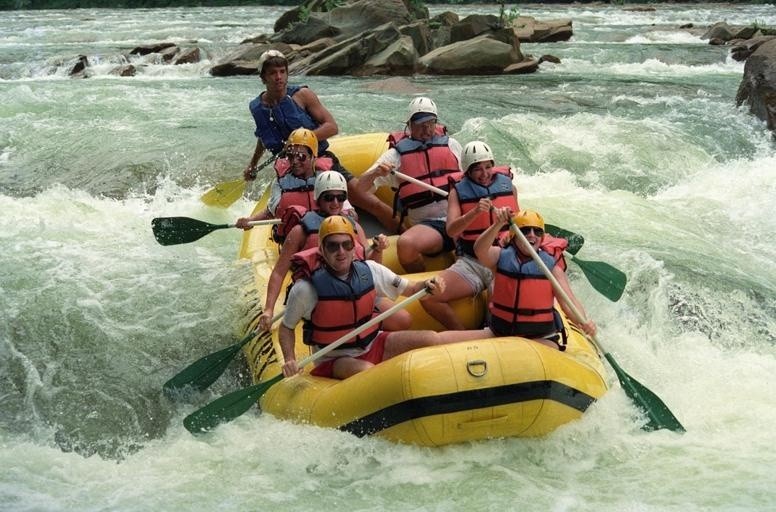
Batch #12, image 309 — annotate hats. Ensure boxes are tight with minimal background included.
[257,50,285,77]
[413,113,436,124]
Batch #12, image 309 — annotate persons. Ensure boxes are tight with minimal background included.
[419,141,518,331]
[243,50,406,231]
[259,170,389,329]
[237,128,357,229]
[438,207,597,351]
[360,96,464,274]
[279,215,445,379]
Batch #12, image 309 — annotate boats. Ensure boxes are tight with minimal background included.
[232,128,609,450]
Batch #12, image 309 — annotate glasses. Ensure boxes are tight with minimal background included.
[519,227,544,238]
[284,153,310,162]
[325,240,354,253]
[319,193,349,203]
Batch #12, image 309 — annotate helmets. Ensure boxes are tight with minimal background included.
[283,126,319,159]
[509,209,545,242]
[460,141,494,174]
[313,170,349,203]
[406,96,437,126]
[317,215,356,248]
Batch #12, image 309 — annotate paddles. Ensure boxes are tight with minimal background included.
[151,217,283,246]
[201,151,281,209]
[563,250,627,302]
[183,282,435,439]
[386,166,584,258]
[509,213,685,430]
[164,310,290,395]
